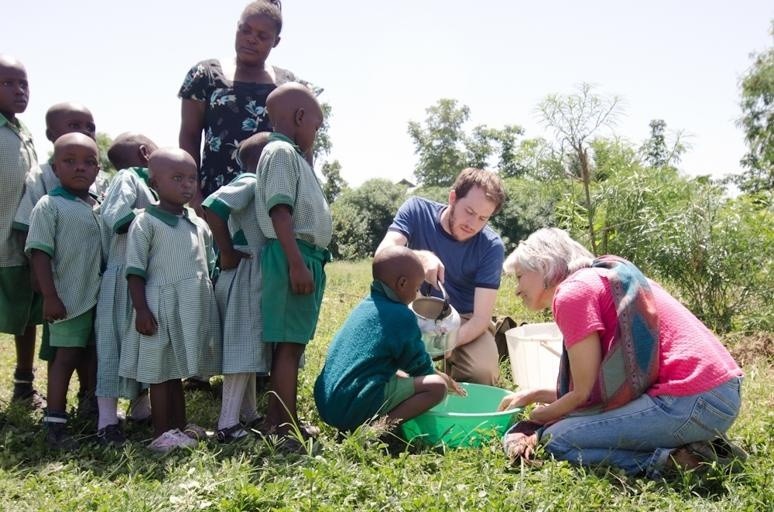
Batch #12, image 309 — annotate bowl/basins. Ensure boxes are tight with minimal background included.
[400,379,524,449]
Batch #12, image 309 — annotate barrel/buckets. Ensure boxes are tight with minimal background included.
[504,322,564,394]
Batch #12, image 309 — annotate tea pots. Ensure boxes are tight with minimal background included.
[407,279,464,353]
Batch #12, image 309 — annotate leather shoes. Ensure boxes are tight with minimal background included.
[217,423,252,444]
[242,415,269,437]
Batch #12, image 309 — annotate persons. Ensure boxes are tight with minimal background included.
[117,147,222,451]
[256,82,333,457]
[312,245,469,461]
[374,168,506,387]
[498,228,746,491]
[24,133,104,440]
[201,133,305,448]
[91,134,155,445]
[0,60,45,407]
[10,102,109,415]
[178,0,304,391]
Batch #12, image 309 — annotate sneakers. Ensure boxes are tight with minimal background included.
[97,420,124,449]
[182,423,208,443]
[280,437,321,453]
[688,436,751,474]
[11,388,46,411]
[46,421,79,449]
[145,427,195,453]
[295,423,321,441]
